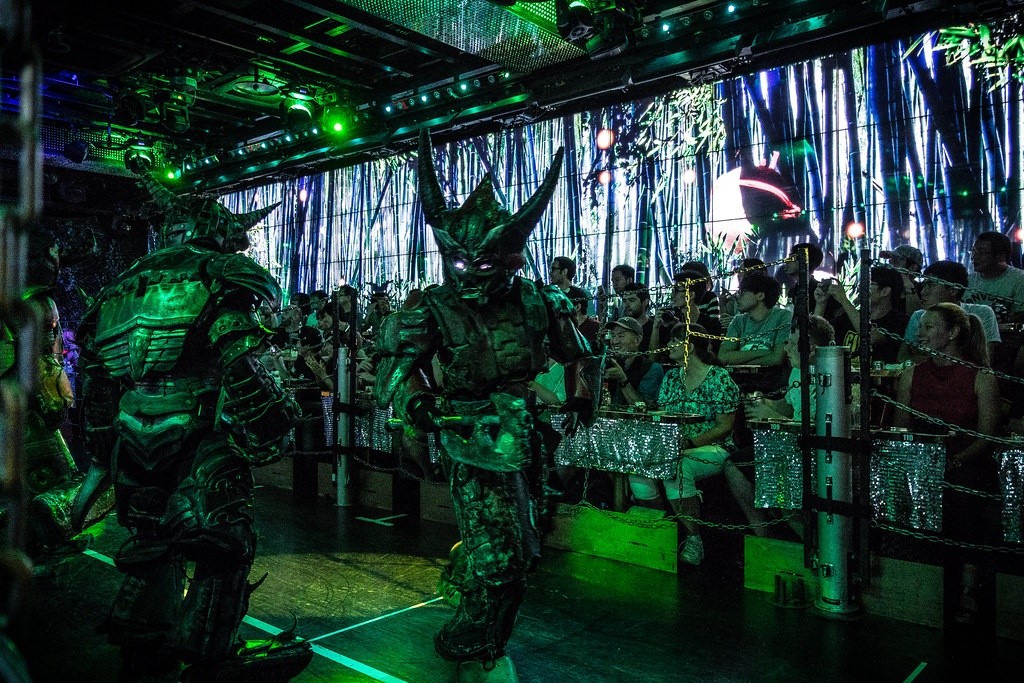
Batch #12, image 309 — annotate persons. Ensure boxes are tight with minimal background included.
[721,257,768,334]
[649,271,724,364]
[891,303,1000,623]
[551,256,576,288]
[785,243,843,319]
[878,245,923,329]
[73,156,307,683]
[958,231,1023,322]
[375,124,597,666]
[621,282,667,363]
[612,265,635,296]
[598,316,663,411]
[259,284,440,390]
[897,261,1002,370]
[724,315,860,544]
[563,286,602,372]
[682,262,720,317]
[630,323,737,565]
[717,273,792,365]
[813,266,905,366]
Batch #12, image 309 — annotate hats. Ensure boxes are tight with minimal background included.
[879,244,924,269]
[289,326,323,342]
[604,316,643,340]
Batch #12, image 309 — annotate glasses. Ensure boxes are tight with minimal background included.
[672,287,686,294]
[550,267,561,273]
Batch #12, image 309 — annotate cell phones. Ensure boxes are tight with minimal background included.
[821,279,832,289]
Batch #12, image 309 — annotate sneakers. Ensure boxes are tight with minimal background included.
[678,534,703,566]
